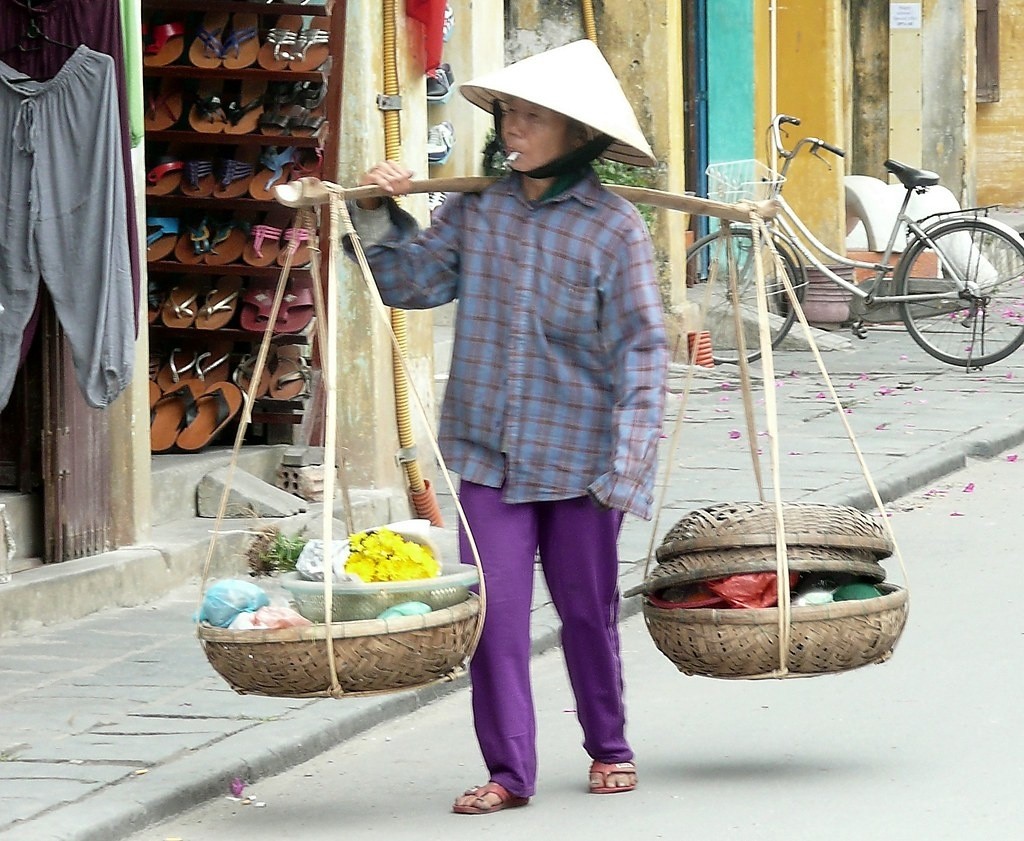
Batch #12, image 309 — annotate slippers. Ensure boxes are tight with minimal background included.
[136,2,336,458]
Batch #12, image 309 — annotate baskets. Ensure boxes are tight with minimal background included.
[197,590,480,699]
[655,500,895,560]
[642,582,909,681]
[623,545,887,599]
[280,561,478,623]
[704,159,788,205]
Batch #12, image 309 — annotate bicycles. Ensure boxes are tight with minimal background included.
[687,113,1024,367]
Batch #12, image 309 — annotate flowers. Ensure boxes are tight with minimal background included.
[345,528,440,586]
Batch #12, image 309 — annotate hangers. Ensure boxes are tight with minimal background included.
[1,0,113,84]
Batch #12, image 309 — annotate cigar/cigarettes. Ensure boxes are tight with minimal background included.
[505,149,520,163]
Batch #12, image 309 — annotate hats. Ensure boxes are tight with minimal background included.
[458,39,661,169]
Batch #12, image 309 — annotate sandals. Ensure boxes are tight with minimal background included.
[453,781,530,815]
[588,760,638,794]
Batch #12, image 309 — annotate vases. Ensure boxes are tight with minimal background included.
[785,263,859,324]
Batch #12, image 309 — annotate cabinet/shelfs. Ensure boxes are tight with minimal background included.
[139,2,339,455]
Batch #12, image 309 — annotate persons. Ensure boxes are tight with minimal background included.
[338,41,668,816]
